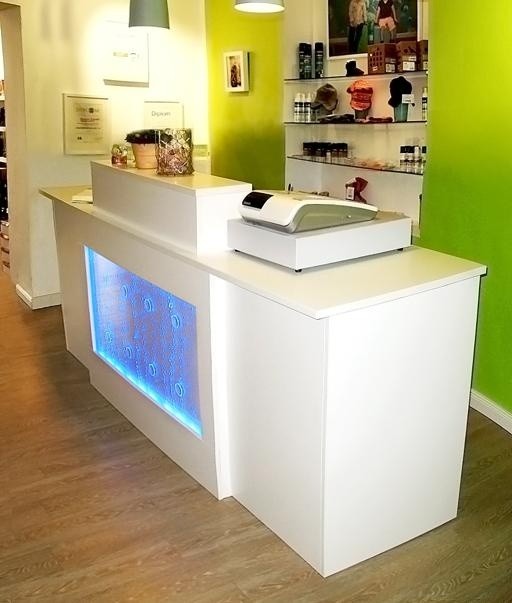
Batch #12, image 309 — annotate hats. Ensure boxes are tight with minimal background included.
[311,84,338,111]
[388,76,412,108]
[347,80,373,111]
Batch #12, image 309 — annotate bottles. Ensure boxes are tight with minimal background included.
[422,88,428,121]
[302,141,348,162]
[400,145,427,167]
[298,41,324,79]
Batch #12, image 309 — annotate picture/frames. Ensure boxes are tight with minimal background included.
[325,0,423,61]
[62,21,185,156]
[222,49,248,93]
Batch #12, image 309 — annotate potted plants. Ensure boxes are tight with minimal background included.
[127,129,160,169]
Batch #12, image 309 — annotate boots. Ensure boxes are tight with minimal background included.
[346,61,364,77]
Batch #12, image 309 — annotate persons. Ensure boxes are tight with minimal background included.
[348,0,367,53]
[375,0,398,43]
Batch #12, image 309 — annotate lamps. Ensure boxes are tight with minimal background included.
[126,0,286,34]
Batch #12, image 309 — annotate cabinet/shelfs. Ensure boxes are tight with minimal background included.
[38,160,487,579]
[1,221,10,274]
[283,69,428,238]
